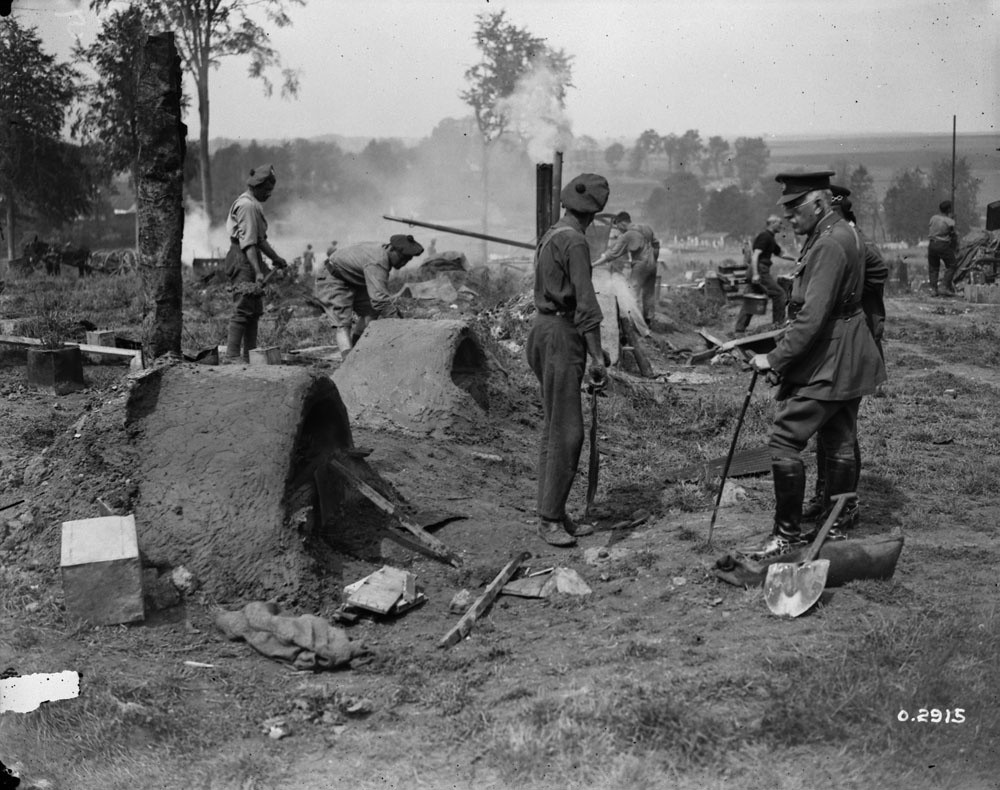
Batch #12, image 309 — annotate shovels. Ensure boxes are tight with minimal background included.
[763,492,860,619]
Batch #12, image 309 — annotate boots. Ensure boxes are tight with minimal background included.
[747,438,861,562]
[801,440,861,520]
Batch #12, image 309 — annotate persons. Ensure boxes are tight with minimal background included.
[428,238,436,257]
[326,240,337,257]
[304,245,316,273]
[733,171,891,562]
[927,200,959,298]
[222,165,288,366]
[526,173,612,546]
[317,234,426,362]
[592,211,660,329]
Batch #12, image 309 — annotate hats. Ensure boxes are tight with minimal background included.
[830,184,851,203]
[775,171,835,207]
[562,173,610,215]
[246,164,274,188]
[613,212,631,225]
[390,233,424,256]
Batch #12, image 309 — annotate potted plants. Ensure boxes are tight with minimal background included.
[27,329,83,394]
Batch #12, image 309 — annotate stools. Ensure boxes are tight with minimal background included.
[58,513,145,624]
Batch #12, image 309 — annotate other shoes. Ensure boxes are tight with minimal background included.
[223,355,250,364]
[537,519,576,545]
[563,516,594,536]
[931,288,938,297]
[774,320,791,328]
[940,287,952,295]
